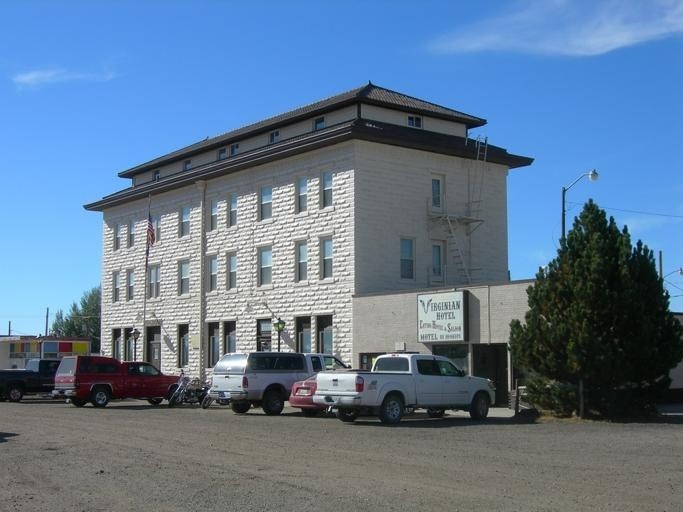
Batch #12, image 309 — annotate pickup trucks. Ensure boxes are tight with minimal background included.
[210,352,496,424]
[0,355,189,407]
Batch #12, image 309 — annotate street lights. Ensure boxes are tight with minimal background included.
[561,168,601,234]
[130,329,141,361]
[658,250,683,279]
[273,317,285,352]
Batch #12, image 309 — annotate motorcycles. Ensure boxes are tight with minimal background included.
[168,369,229,409]
